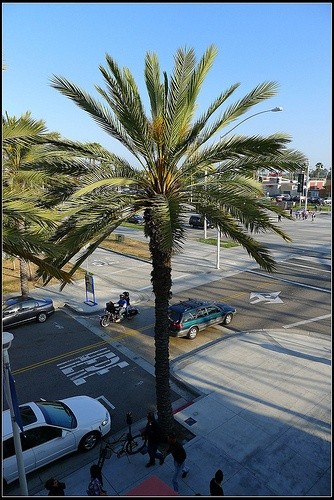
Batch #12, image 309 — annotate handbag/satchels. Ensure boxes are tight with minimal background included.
[140,446,148,456]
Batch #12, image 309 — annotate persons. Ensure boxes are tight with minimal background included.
[88,464,103,495]
[278,215,281,221]
[210,470,224,496]
[115,294,127,323]
[312,212,315,221]
[302,211,304,220]
[290,208,292,217]
[45,479,65,496]
[142,411,165,467]
[157,433,190,493]
[123,292,130,319]
[315,205,318,211]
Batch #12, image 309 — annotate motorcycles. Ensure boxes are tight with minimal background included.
[99,301,139,327]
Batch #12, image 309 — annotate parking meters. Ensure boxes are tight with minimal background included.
[126,411,133,452]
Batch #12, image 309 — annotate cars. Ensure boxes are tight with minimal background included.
[265,193,331,205]
[3,294,55,331]
[2,394,111,490]
[188,214,214,230]
[126,214,144,224]
[167,298,236,340]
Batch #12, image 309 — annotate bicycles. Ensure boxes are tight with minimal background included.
[97,432,147,473]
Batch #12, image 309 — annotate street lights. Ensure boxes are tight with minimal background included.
[215,106,283,268]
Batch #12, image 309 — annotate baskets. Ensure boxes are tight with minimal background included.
[100,448,111,459]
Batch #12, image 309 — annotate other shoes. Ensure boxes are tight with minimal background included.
[159,455,166,465]
[182,472,187,478]
[146,460,155,467]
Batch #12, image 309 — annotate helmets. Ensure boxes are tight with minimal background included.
[123,292,129,296]
[120,294,125,298]
